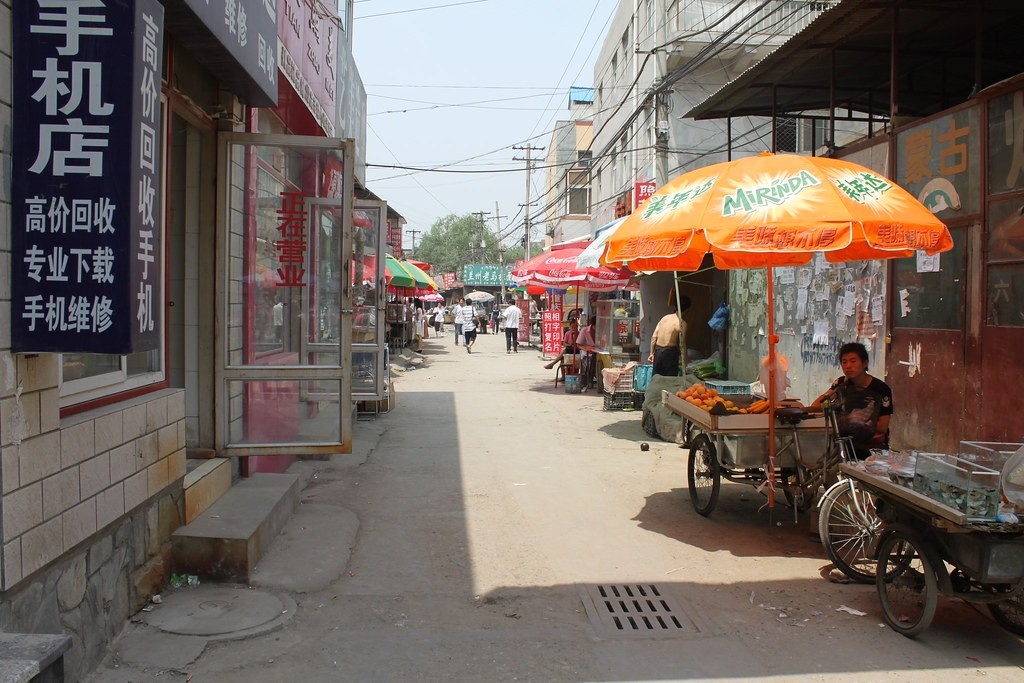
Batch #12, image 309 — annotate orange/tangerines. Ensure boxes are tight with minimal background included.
[676,384,746,415]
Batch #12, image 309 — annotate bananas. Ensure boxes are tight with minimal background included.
[747,397,770,414]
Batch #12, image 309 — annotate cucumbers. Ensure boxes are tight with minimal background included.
[693,364,719,378]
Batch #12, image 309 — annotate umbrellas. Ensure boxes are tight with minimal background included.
[350,250,444,336]
[508,215,640,374]
[599,150,954,510]
[463,291,495,308]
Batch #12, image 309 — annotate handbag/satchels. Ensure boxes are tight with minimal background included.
[708,306,729,331]
[472,316,479,325]
[490,320,493,328]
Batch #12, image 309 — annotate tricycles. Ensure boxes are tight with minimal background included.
[658,381,920,586]
[854,480,1024,640]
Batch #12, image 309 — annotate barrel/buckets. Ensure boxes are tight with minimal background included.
[565,374,581,394]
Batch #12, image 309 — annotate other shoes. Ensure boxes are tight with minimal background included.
[507,350,510,354]
[435,331,438,338]
[440,332,446,337]
[414,350,422,353]
[467,346,471,354]
[544,364,552,369]
[559,378,564,382]
[514,351,518,353]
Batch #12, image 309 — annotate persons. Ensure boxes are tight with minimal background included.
[811,343,894,509]
[648,295,691,378]
[544,318,580,382]
[576,316,604,393]
[405,296,552,353]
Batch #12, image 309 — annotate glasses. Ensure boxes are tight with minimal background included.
[507,302,510,304]
